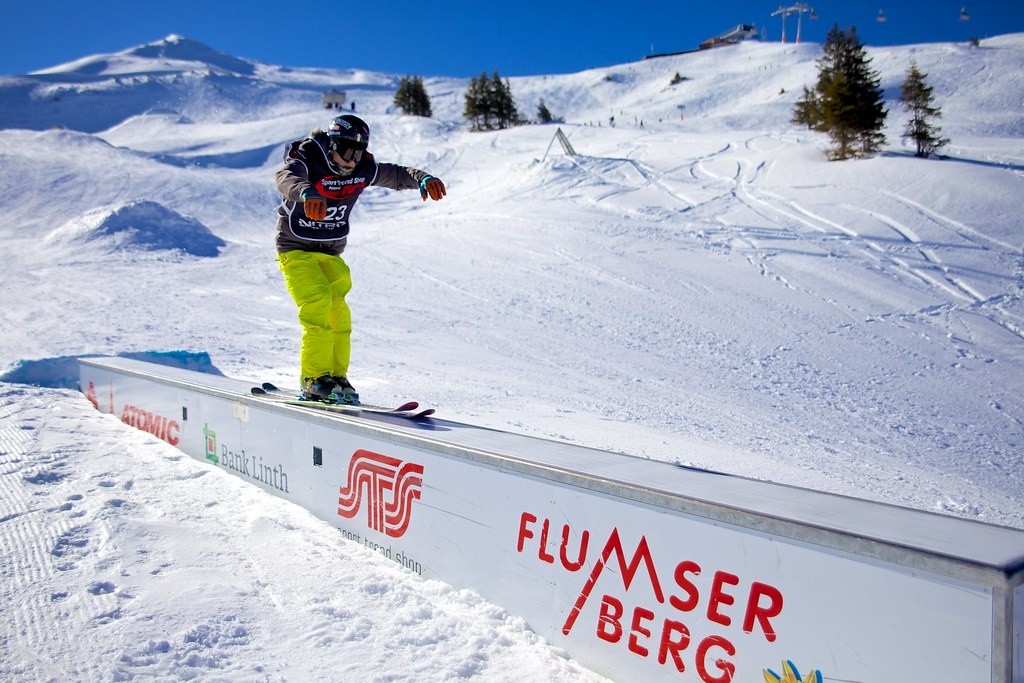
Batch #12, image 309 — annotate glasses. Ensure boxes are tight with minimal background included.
[328,143,363,163]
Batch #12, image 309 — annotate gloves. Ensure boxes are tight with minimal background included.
[420,176,447,200]
[301,188,327,220]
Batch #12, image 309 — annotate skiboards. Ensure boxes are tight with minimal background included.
[250,382,435,420]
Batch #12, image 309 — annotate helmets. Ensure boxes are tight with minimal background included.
[328,115,369,146]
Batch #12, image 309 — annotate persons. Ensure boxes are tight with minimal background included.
[274,114,446,392]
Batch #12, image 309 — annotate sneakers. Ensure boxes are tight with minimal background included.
[331,376,359,401]
[301,375,343,399]
